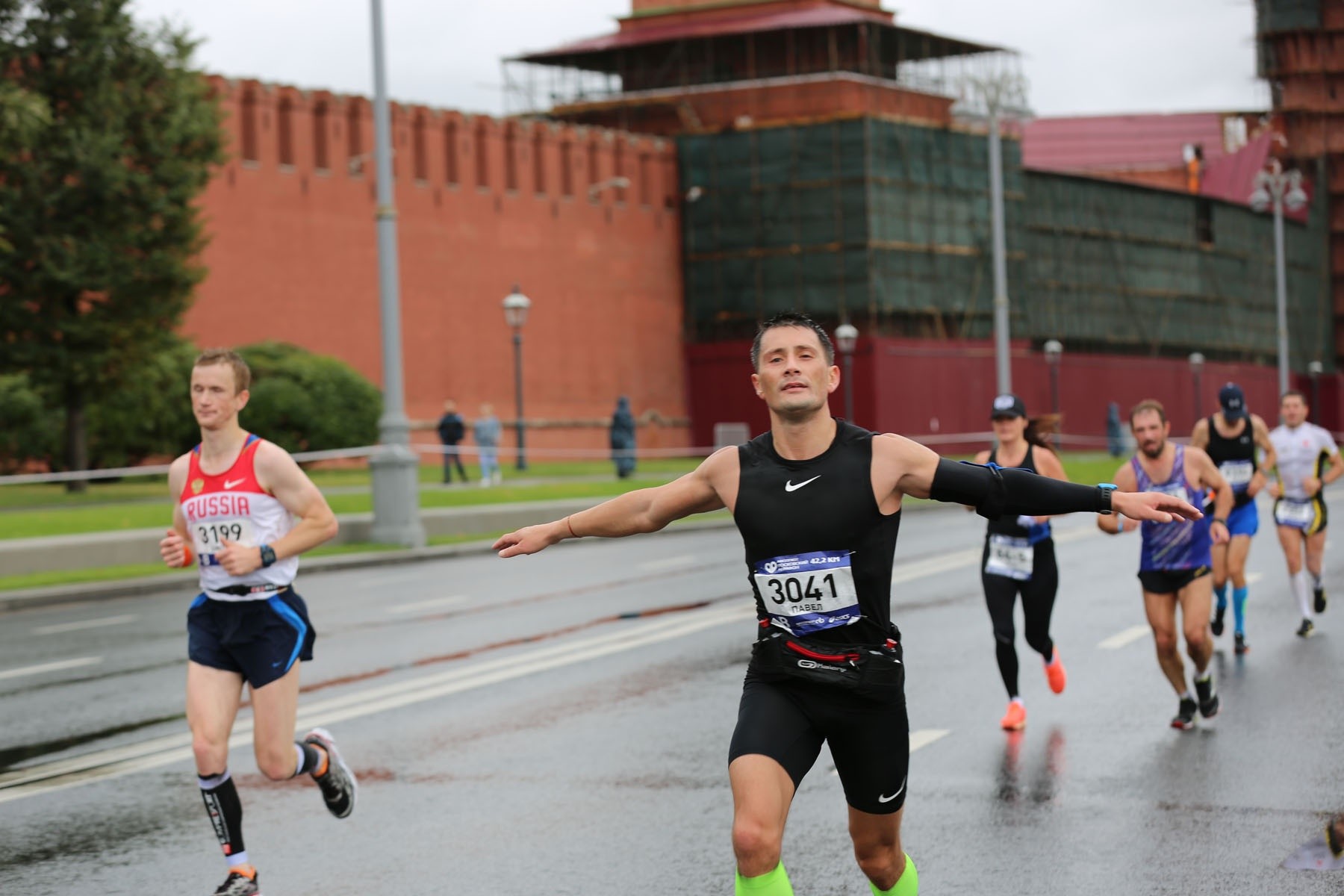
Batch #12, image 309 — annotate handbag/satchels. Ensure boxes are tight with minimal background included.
[754,632,872,693]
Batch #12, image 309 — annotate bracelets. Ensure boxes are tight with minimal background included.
[568,516,583,538]
[1213,517,1227,527]
[1319,477,1325,489]
[182,546,194,568]
[1260,464,1269,478]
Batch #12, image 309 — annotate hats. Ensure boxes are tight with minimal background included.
[1219,382,1246,421]
[988,393,1026,420]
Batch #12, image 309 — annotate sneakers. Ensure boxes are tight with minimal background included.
[303,727,357,818]
[210,865,260,896]
[1193,672,1219,717]
[1170,698,1197,729]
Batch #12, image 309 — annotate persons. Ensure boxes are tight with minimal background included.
[969,380,1343,733]
[494,311,1207,896]
[437,398,639,488]
[156,352,359,896]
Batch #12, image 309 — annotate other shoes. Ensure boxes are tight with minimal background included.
[1296,619,1315,638]
[1233,632,1247,654]
[1209,596,1227,636]
[1044,646,1065,694]
[1001,701,1027,729]
[1313,583,1326,613]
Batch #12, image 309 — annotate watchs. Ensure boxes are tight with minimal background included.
[259,544,277,566]
[1096,482,1118,515]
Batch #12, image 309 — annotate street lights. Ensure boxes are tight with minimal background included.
[944,72,1036,413]
[1249,156,1306,425]
[833,324,861,431]
[1188,352,1206,430]
[1043,339,1063,450]
[1309,360,1326,425]
[502,294,533,469]
